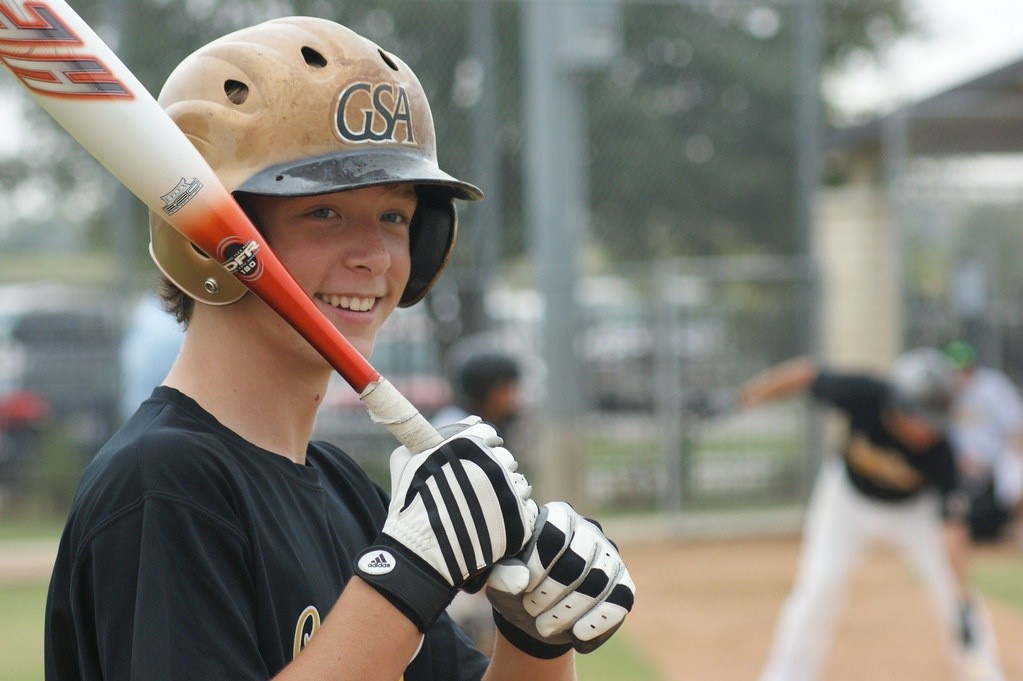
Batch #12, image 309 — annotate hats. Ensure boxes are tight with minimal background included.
[458,352,518,401]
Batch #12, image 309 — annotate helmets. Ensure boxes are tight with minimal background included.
[148,16,483,308]
[890,348,967,427]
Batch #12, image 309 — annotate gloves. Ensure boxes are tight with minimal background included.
[354,415,540,634]
[486,502,637,659]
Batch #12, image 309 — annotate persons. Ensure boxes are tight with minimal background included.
[429,354,522,658]
[942,341,1023,541]
[44,15,638,681]
[737,347,1003,681]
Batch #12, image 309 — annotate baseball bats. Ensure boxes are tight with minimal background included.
[0,0,447,455]
